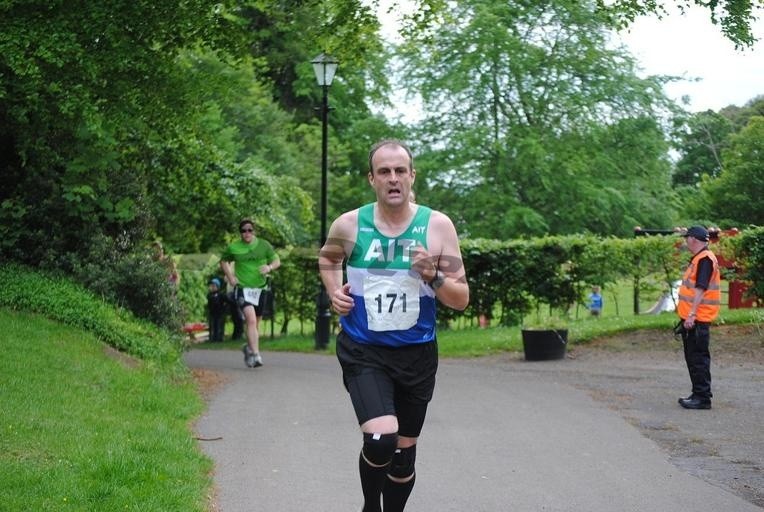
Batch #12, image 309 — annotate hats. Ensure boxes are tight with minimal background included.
[680,226,710,243]
[207,278,221,288]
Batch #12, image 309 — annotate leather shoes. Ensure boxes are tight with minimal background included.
[678,395,711,409]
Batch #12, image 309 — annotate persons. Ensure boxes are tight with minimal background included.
[318,140,470,512]
[676,225,721,409]
[586,286,603,318]
[204,220,281,369]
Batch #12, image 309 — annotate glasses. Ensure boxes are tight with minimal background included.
[239,228,253,233]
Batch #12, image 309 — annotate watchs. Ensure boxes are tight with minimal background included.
[688,311,697,317]
[428,270,445,289]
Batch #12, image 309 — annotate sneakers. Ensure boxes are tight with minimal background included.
[253,352,263,368]
[242,343,254,367]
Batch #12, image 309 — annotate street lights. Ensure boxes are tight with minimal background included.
[305,51,339,349]
